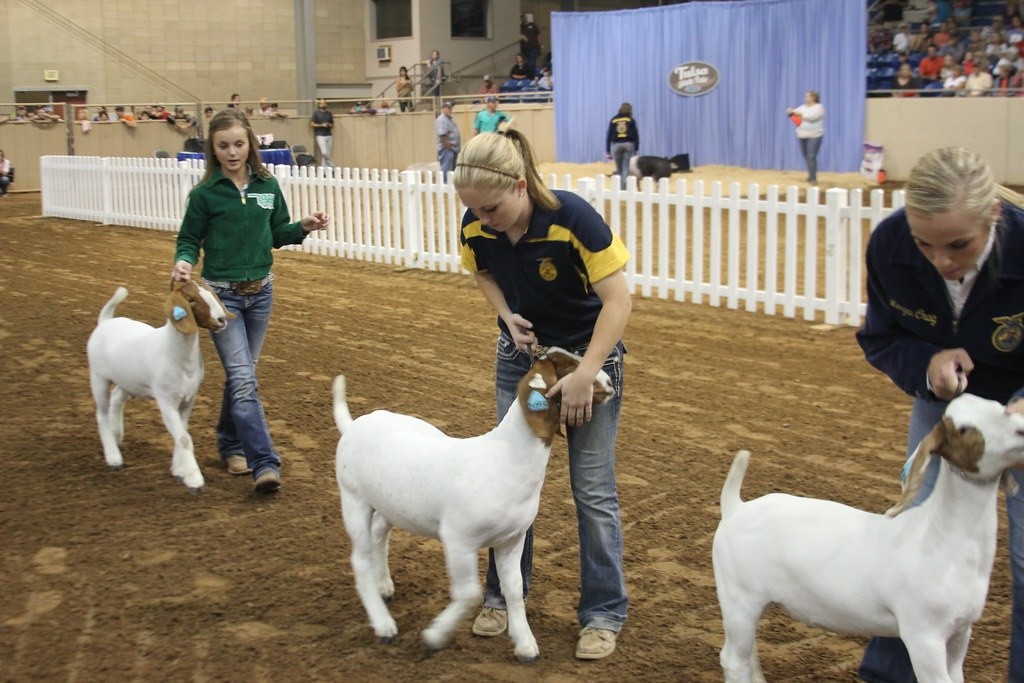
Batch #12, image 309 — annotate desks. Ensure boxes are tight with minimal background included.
[177,148,293,176]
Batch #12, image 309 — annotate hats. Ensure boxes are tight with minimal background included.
[443,102,454,107]
[485,96,500,103]
[483,75,491,82]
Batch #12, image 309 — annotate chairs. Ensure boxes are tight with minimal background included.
[865,3,1007,98]
[153,150,170,158]
[471,56,553,103]
[292,145,317,169]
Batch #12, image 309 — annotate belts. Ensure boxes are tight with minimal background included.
[203,272,274,295]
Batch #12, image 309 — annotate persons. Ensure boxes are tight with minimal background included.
[510,53,531,80]
[519,15,541,69]
[395,67,415,113]
[455,124,633,660]
[228,94,241,110]
[434,101,461,184]
[472,96,511,137]
[309,98,336,169]
[0,105,63,124]
[257,98,288,119]
[534,52,553,102]
[865,0,1024,97]
[605,102,639,190]
[787,91,826,185]
[855,143,1024,683]
[420,50,445,95]
[350,100,397,114]
[174,108,332,492]
[0,150,10,198]
[478,75,500,104]
[77,104,213,129]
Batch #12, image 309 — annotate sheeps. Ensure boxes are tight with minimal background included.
[711,392,1024,683]
[86,272,238,489]
[330,346,618,666]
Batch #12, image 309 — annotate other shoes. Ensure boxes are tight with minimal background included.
[471,604,507,635]
[575,626,618,659]
[810,179,817,187]
[223,454,253,475]
[253,468,280,493]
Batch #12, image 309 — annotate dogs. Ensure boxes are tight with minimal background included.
[628,155,679,187]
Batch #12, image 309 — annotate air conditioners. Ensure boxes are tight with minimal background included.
[521,13,534,24]
[376,47,390,61]
